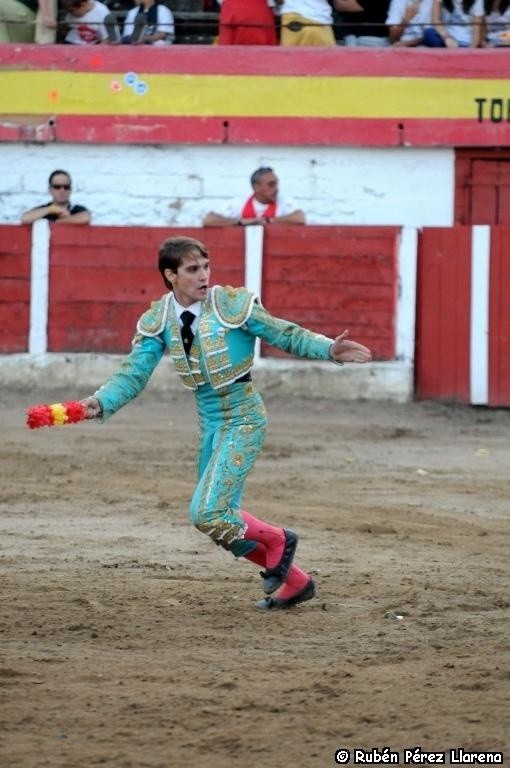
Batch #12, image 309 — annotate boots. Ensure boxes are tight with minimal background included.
[241,540,316,611]
[238,507,298,595]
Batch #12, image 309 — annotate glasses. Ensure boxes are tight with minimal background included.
[50,184,71,190]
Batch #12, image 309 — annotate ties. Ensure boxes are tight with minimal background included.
[180,310,197,355]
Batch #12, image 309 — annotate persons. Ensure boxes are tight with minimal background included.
[202,163,306,229]
[74,234,374,610]
[20,168,93,227]
[2,1,509,48]
